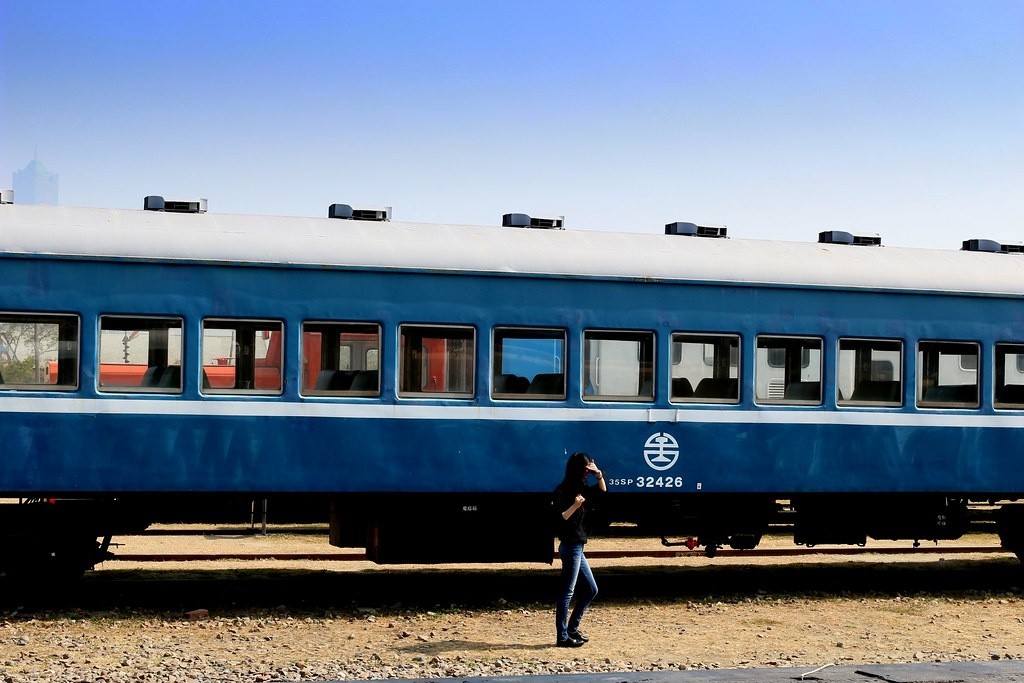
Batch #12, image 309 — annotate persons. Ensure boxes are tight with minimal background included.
[555,453,612,647]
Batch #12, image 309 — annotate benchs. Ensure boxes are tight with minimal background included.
[924,384,978,404]
[784,382,821,400]
[493,372,564,395]
[995,384,1024,404]
[641,377,739,398]
[848,381,901,402]
[140,365,211,390]
[313,369,379,391]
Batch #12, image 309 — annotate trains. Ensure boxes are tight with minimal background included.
[0,190,1024,575]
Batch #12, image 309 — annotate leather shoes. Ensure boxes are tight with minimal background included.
[567,626,589,642]
[556,637,584,648]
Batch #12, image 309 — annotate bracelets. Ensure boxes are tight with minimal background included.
[598,476,603,481]
